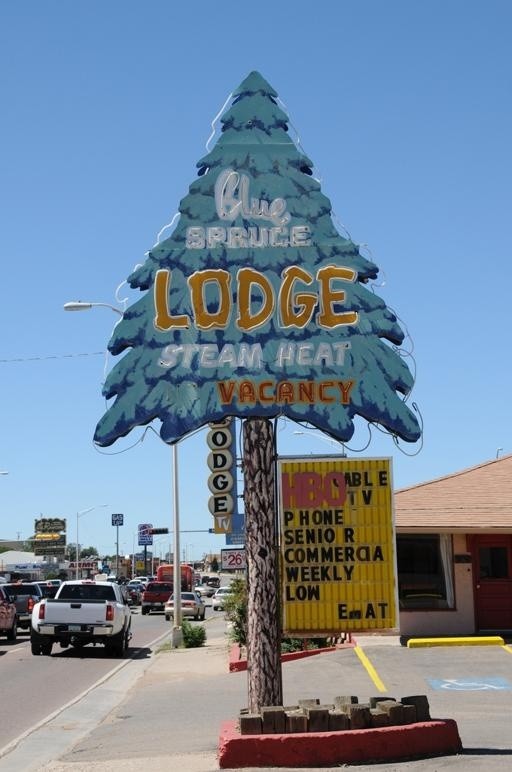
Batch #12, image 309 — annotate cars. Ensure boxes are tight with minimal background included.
[0,562,237,656]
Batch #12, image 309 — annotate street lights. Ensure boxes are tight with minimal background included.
[1,470,10,476]
[64,301,185,650]
[77,503,108,579]
[292,429,347,457]
[496,448,503,459]
[131,527,154,579]
